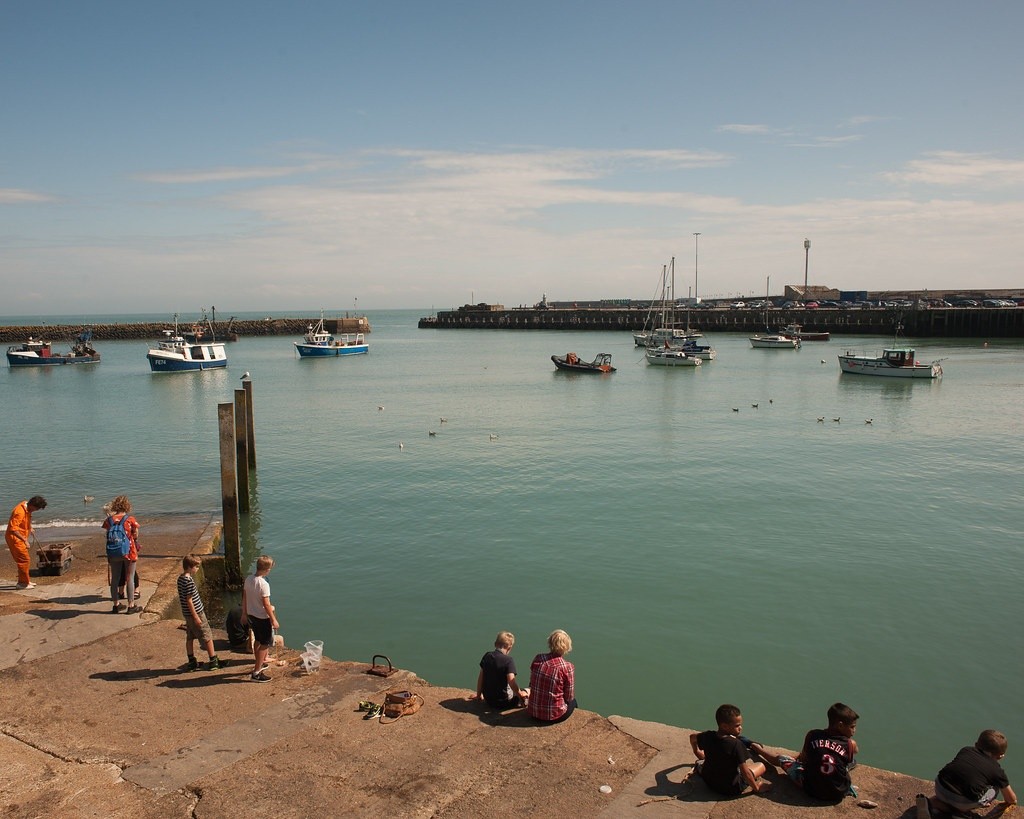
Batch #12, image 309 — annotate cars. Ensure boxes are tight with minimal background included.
[701,303,715,309]
[637,303,649,309]
[671,303,685,309]
[782,298,1017,308]
[729,300,774,310]
[537,304,549,310]
[690,303,700,309]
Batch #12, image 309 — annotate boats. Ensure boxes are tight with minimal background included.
[293,308,369,358]
[6,323,101,367]
[551,352,618,373]
[146,306,239,374]
[748,277,830,348]
[837,304,943,377]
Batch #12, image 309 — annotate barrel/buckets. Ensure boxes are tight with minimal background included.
[300,650,321,675]
[304,640,324,660]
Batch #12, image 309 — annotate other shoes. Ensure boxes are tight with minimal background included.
[126,603,143,613]
[113,602,127,613]
[188,657,197,672]
[110,593,124,600]
[261,663,269,670]
[847,760,857,771]
[30,582,37,586]
[251,671,272,682]
[209,654,219,670]
[134,591,141,599]
[916,794,933,819]
[16,583,35,589]
[736,736,764,752]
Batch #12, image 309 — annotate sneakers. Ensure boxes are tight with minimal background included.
[359,700,375,712]
[364,704,382,720]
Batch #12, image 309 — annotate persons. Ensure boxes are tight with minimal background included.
[240,555,279,683]
[528,630,578,724]
[689,704,772,796]
[177,555,229,672]
[751,703,859,798]
[226,587,252,645]
[936,730,1017,811]
[5,495,47,589]
[469,631,530,710]
[102,495,143,614]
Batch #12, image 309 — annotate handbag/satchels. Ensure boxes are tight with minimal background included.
[380,690,424,724]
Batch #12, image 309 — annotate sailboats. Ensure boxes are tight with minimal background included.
[632,258,716,366]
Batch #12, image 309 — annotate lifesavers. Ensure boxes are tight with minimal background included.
[328,340,332,345]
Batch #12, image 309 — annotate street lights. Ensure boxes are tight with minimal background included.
[693,233,701,297]
[804,237,811,303]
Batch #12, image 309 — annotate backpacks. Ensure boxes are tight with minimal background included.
[106,514,130,561]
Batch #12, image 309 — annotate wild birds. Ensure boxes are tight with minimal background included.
[378,405,384,410]
[429,431,436,435]
[770,399,773,402]
[440,417,448,422]
[865,419,874,423]
[833,417,841,421]
[821,359,826,363]
[732,408,739,411]
[490,433,499,439]
[817,417,826,420]
[399,442,404,447]
[85,495,95,502]
[752,404,758,407]
